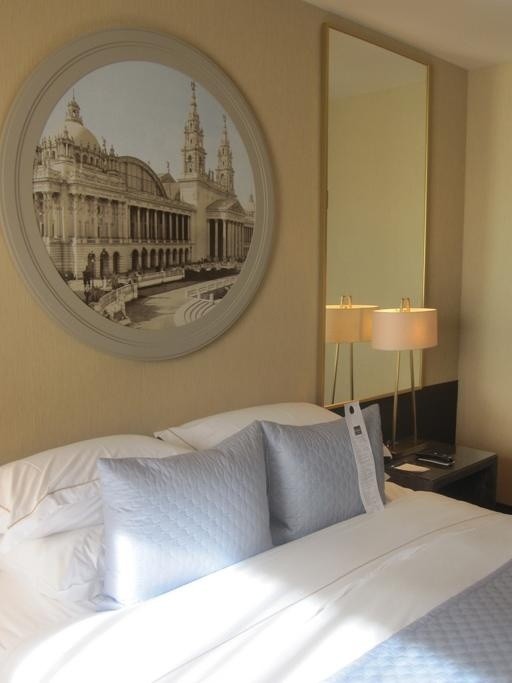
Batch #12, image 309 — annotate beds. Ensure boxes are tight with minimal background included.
[1,481,512,681]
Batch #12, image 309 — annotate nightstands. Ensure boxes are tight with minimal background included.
[385,438,498,512]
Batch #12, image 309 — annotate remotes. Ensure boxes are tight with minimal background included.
[418,457,452,469]
[416,451,448,460]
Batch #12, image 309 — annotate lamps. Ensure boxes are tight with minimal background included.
[326,294,380,405]
[373,297,439,461]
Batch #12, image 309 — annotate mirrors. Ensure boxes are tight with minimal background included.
[317,20,431,408]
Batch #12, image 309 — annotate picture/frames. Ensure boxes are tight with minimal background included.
[0,25,278,363]
